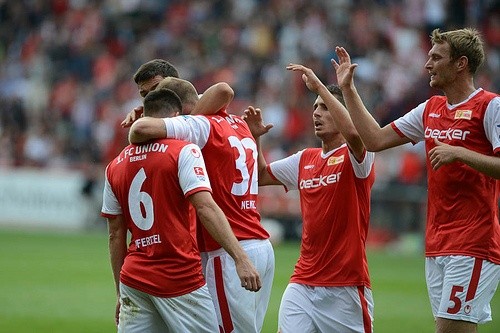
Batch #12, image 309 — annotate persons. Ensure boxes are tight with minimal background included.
[100,57,275,333]
[330,27,500,333]
[0,0,500,181]
[242,63,376,333]
